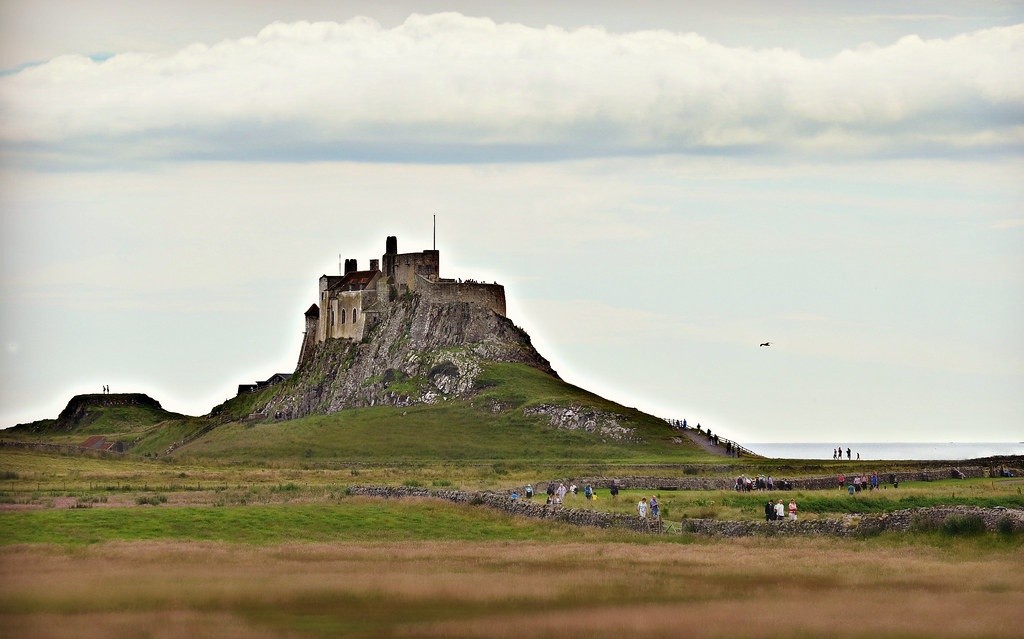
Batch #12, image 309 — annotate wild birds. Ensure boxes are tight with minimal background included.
[759,342,770,347]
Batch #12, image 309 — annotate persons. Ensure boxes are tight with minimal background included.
[650,495,659,518]
[755,474,773,491]
[638,497,648,518]
[697,423,701,435]
[585,484,598,500]
[103,385,109,394]
[707,429,719,445]
[611,484,618,496]
[848,472,878,494]
[526,483,533,498]
[1004,470,1013,477]
[726,441,740,458]
[834,447,860,460]
[512,492,517,499]
[765,500,784,521]
[893,475,898,488]
[839,474,844,487]
[547,483,577,512]
[789,499,797,521]
[736,474,752,492]
[676,419,687,429]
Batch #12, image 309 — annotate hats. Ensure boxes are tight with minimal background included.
[527,484,531,487]
